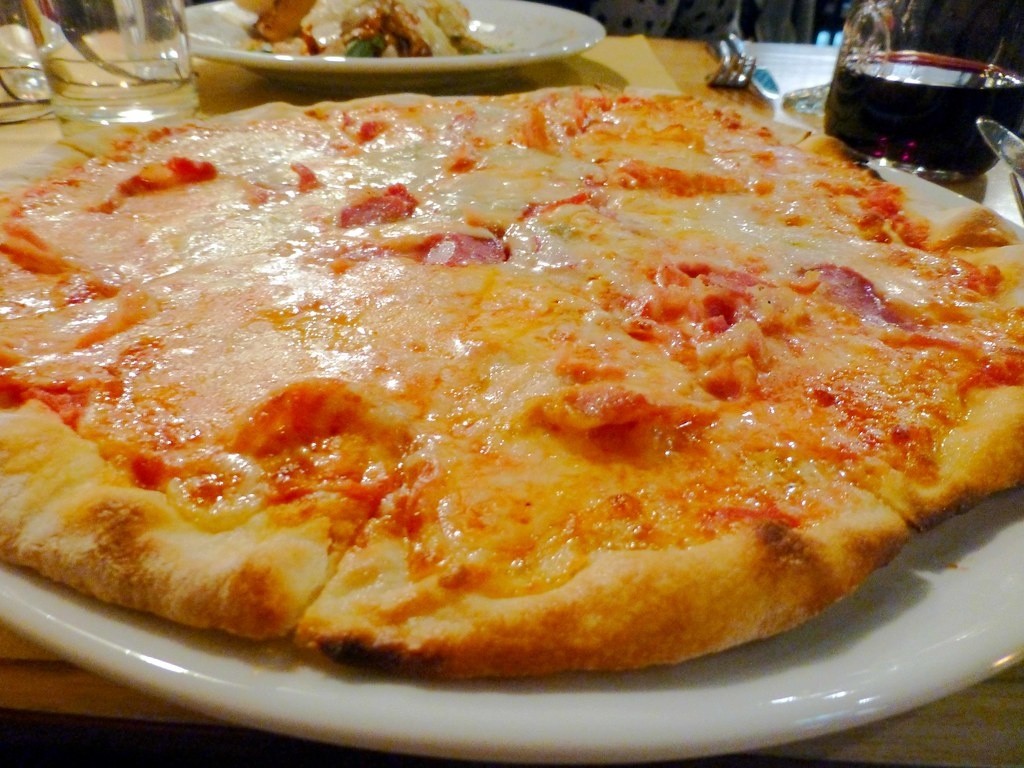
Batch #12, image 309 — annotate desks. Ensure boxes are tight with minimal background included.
[0,36,1024,768]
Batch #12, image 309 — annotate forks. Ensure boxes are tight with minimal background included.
[705,42,756,88]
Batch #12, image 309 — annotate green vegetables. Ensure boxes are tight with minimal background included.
[346,36,386,57]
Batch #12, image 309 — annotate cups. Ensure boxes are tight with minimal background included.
[823,0,1024,182]
[0,0,204,124]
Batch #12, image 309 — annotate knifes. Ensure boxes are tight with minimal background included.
[727,33,781,100]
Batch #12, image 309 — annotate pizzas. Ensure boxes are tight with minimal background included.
[0,85,1024,674]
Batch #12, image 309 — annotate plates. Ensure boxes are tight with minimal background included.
[185,0,606,92]
[0,156,1024,764]
